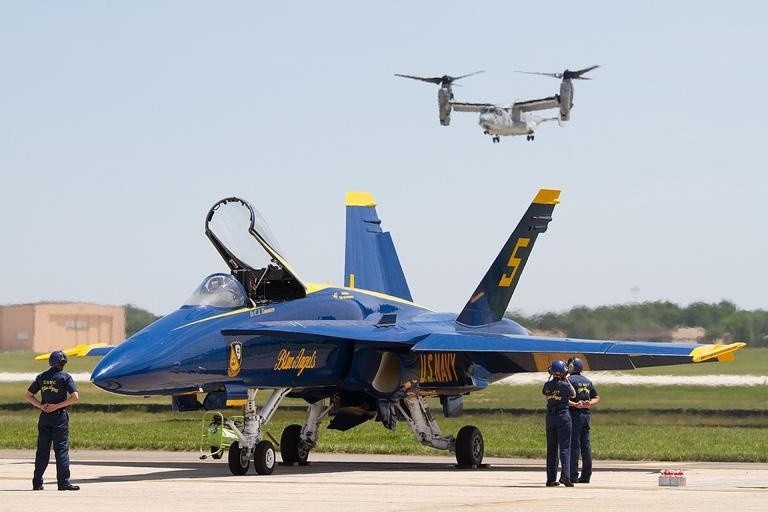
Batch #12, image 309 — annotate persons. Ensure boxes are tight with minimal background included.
[24,348,80,491]
[565,356,600,483]
[541,360,577,488]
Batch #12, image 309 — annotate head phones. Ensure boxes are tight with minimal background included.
[60,351,66,365]
[568,358,576,374]
[547,360,568,376]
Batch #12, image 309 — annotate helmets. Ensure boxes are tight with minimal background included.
[548,359,567,374]
[567,357,584,374]
[48,350,68,366]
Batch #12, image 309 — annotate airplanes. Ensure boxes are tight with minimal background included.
[394,65,600,140]
[34,188,747,475]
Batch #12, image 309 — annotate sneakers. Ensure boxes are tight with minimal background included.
[57,484,80,491]
[33,485,44,490]
[545,480,559,486]
[559,478,574,487]
